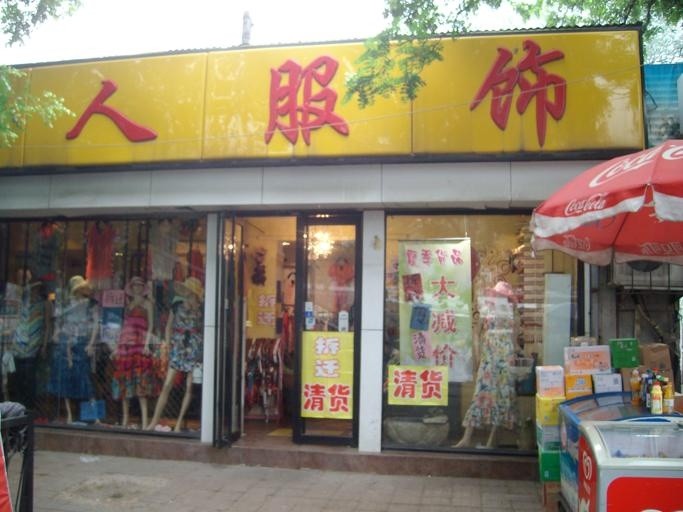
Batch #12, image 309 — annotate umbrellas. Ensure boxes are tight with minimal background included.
[527,138,683,267]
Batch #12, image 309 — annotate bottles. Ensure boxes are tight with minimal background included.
[629,366,675,415]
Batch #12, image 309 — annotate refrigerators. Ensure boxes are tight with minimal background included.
[556,389,682,512]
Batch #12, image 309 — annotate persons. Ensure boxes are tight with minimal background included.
[47,274,107,427]
[110,275,158,431]
[7,278,51,416]
[144,275,203,434]
[450,281,523,449]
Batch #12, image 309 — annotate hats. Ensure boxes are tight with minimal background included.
[173,277,203,300]
[484,281,521,307]
[69,275,90,295]
[124,275,151,298]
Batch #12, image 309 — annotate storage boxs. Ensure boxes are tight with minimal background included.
[565,335,675,402]
[534,365,565,509]
